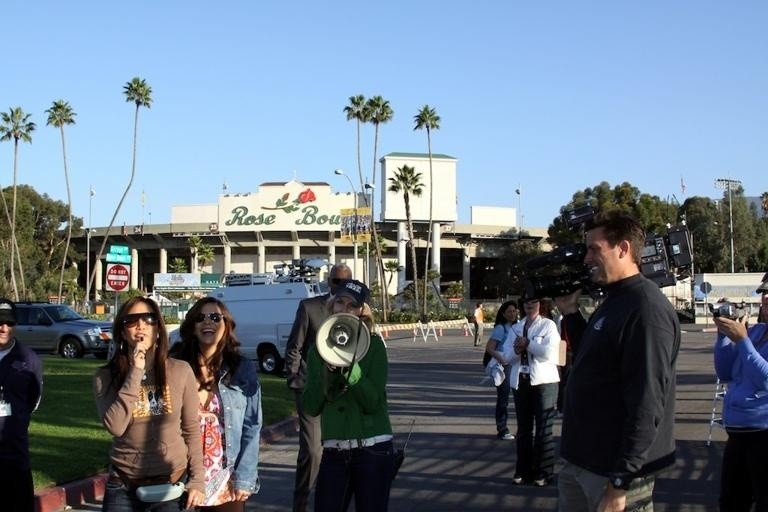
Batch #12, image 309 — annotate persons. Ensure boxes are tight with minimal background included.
[92,295,206,512]
[165,297,264,512]
[551,209,682,512]
[712,277,768,511]
[284,264,372,512]
[0,297,43,512]
[472,302,483,347]
[479,296,588,486]
[303,280,394,512]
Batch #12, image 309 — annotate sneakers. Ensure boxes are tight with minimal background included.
[511,477,522,485]
[532,478,547,487]
[501,434,514,440]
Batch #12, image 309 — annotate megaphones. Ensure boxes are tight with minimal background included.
[315,312,371,368]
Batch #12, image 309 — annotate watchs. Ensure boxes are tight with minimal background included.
[609,475,629,491]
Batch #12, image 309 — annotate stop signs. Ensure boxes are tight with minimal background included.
[106,264,130,292]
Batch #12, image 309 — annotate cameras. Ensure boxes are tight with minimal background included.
[519,365,530,374]
[707,301,751,320]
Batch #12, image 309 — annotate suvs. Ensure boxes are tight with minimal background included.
[1,301,116,359]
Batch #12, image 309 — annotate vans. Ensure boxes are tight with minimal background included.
[168,282,328,375]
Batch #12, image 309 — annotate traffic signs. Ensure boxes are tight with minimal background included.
[106,254,131,263]
[110,245,129,255]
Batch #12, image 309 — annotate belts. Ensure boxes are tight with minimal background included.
[518,373,529,381]
[108,470,186,485]
[322,434,393,450]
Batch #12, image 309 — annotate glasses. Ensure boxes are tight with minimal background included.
[0,319,17,327]
[194,312,224,323]
[122,312,158,328]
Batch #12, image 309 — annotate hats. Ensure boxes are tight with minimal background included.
[1,298,18,321]
[335,278,367,308]
[755,278,767,294]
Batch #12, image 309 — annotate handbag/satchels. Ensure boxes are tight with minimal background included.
[135,481,185,503]
[482,323,505,367]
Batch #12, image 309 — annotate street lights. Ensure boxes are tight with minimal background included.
[88,185,95,227]
[714,175,741,273]
[334,170,376,280]
[515,182,525,301]
[80,226,97,314]
